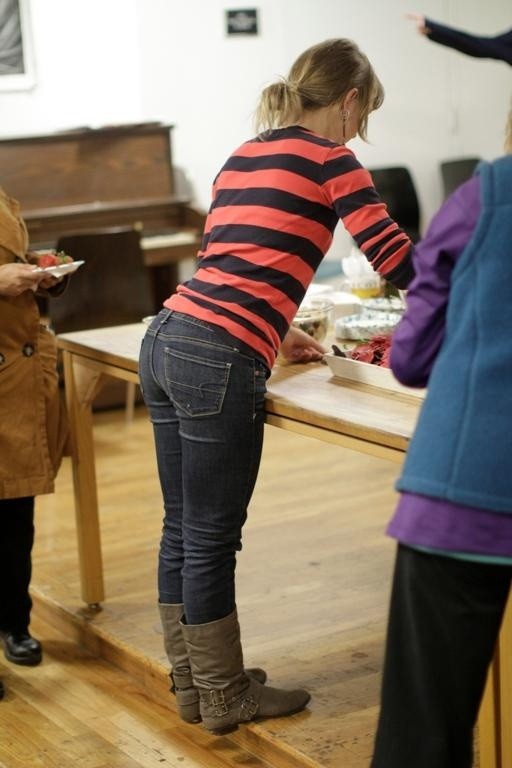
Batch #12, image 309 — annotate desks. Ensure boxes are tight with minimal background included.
[54,322,511,767]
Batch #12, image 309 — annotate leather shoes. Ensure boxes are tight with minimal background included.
[0,630,42,665]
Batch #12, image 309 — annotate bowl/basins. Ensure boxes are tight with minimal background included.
[285,299,334,347]
[344,270,381,302]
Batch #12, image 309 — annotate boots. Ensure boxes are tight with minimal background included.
[157,599,266,723]
[178,604,310,735]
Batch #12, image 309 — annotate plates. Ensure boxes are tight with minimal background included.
[31,261,86,280]
[322,349,430,400]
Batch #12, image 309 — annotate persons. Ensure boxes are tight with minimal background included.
[370,110,511,768]
[405,12,511,65]
[138,38,417,735]
[1,190,72,701]
